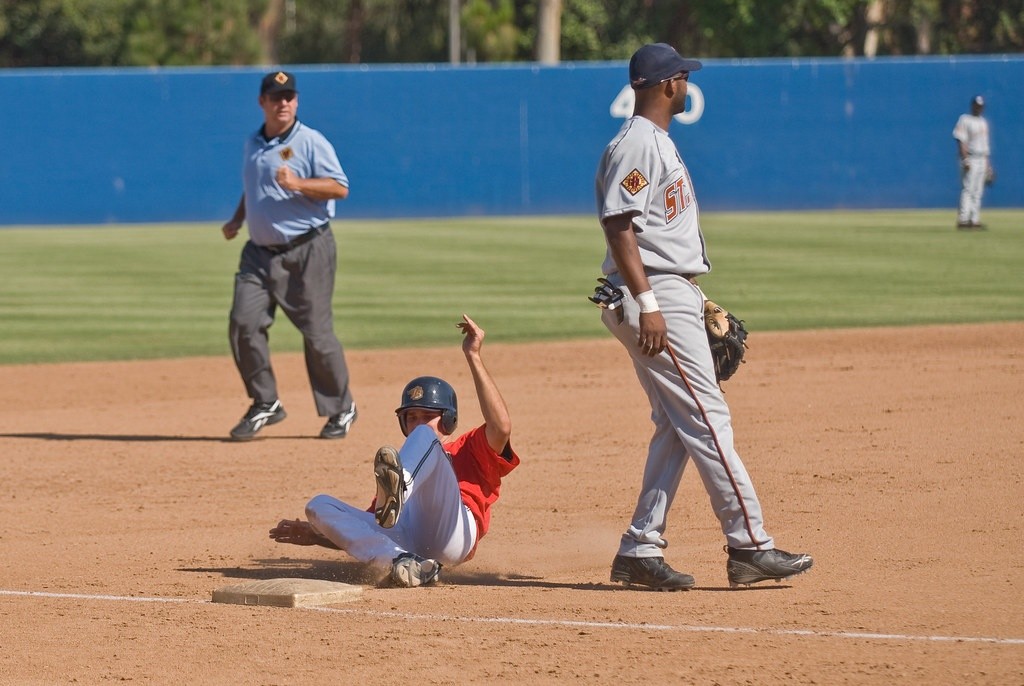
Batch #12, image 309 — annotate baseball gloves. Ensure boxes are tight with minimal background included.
[704,300,748,383]
[986,167,994,188]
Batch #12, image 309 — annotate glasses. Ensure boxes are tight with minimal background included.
[659,72,689,84]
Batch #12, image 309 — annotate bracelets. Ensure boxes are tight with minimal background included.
[696,286,708,300]
[635,289,660,313]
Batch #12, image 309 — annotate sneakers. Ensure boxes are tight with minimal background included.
[231,400,286,437]
[391,557,443,586]
[723,544,813,588]
[320,402,358,438]
[373,445,404,529]
[611,553,695,592]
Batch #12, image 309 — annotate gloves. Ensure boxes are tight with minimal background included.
[588,278,624,309]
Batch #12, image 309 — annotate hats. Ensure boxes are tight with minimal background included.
[629,43,702,89]
[261,71,299,96]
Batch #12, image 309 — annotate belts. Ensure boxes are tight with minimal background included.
[262,223,328,253]
[648,268,698,285]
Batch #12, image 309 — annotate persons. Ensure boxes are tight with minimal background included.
[953,95,993,230]
[269,314,520,589]
[596,43,814,591]
[221,71,358,439]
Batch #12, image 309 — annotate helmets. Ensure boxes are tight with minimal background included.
[395,377,458,438]
